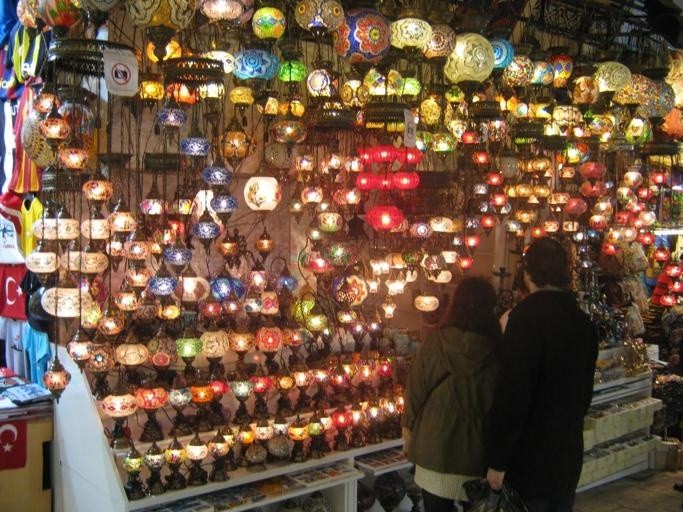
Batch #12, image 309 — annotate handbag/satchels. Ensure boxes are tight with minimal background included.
[464,482,536,511]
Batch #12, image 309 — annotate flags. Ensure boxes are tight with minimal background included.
[0,423,26,468]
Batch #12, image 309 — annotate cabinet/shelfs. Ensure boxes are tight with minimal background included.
[0,411,56,512]
[127,372,664,512]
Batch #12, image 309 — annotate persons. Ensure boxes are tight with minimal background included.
[486,236,599,511]
[401,276,504,511]
